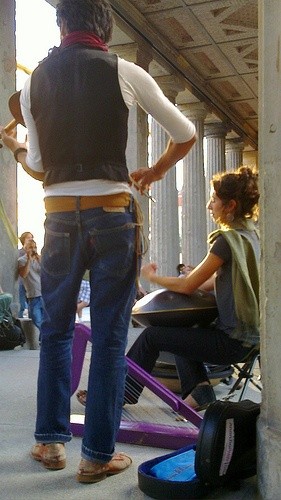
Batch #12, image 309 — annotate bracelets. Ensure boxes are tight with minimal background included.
[14,147,28,162]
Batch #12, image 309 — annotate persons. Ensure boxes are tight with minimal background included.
[75,165,260,416]
[76,269,92,321]
[18,240,43,322]
[18,232,34,319]
[1,1,198,485]
[177,263,187,277]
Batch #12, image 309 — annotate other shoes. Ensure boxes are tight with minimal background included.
[77,453,132,482]
[31,442,67,469]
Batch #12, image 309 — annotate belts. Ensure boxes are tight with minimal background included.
[44,192,130,212]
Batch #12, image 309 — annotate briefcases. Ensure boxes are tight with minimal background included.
[137,400,262,498]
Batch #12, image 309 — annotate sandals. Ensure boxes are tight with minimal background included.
[175,385,220,420]
[76,390,86,406]
